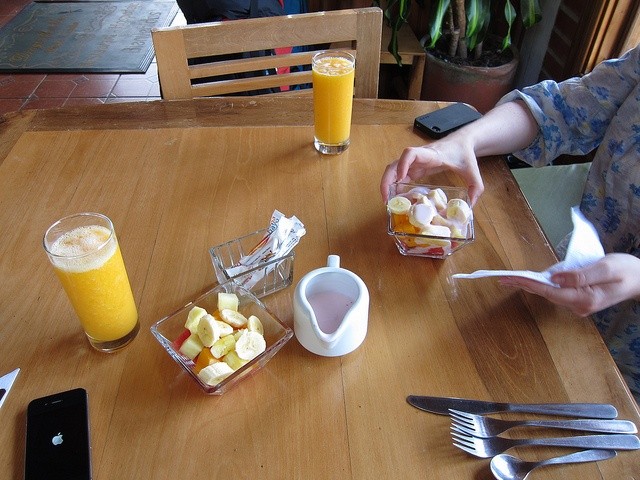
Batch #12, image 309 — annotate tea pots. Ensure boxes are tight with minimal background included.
[291,254,373,359]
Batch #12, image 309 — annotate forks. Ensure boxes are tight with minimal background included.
[448,431,640,458]
[448,408,640,437]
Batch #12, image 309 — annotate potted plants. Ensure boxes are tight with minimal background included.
[373,0,543,113]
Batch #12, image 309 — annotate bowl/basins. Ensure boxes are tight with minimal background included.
[385,182,474,262]
[210,227,295,295]
[148,280,292,397]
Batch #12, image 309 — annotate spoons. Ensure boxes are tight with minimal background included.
[490,449,640,480]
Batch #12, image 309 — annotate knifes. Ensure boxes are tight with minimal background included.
[399,394,619,419]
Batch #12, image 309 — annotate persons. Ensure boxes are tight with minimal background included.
[380,43,640,410]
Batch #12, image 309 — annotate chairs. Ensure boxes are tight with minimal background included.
[151,7,383,98]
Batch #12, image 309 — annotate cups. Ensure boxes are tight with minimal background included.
[311,48,357,156]
[41,212,142,354]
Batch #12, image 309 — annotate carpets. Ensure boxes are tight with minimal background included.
[0,0,179,75]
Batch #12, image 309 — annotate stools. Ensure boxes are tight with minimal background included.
[331,20,426,100]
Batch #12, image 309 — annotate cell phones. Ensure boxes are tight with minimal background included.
[23,388,94,479]
[415,102,486,139]
